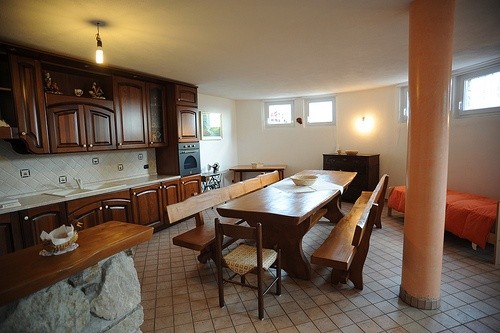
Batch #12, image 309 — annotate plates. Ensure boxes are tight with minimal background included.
[90,97,106,99]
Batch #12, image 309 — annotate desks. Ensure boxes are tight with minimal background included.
[216,170,358,280]
[322,153,380,203]
[229,165,286,184]
[0,221,153,333]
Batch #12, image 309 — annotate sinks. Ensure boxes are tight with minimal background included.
[84,182,126,190]
[44,186,92,198]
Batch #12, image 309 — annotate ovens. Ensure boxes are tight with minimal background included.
[156,142,202,178]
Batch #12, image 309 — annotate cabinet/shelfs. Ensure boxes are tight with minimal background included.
[0,39,201,255]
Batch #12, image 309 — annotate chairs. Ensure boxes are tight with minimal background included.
[215,217,282,320]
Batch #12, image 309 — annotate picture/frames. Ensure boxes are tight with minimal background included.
[199,111,223,140]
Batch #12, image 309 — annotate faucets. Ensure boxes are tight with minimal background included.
[73,177,82,189]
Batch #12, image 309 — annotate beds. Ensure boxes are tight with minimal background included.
[388,185,500,265]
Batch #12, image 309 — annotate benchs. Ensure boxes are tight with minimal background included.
[166,170,389,290]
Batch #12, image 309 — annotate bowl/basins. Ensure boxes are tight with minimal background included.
[290,175,318,186]
[345,150,359,155]
